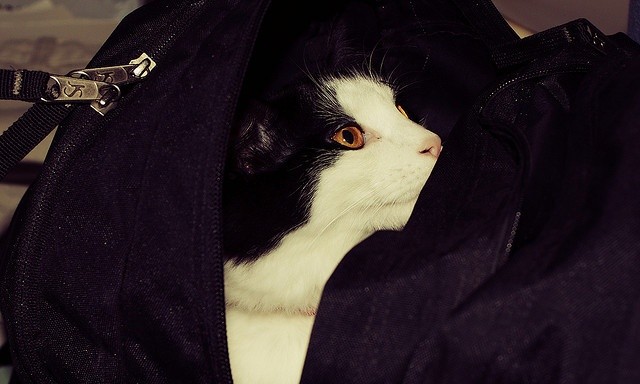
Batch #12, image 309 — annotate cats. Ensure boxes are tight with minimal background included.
[218,42,444,384]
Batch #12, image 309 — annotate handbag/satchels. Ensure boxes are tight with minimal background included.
[0,0,640,383]
